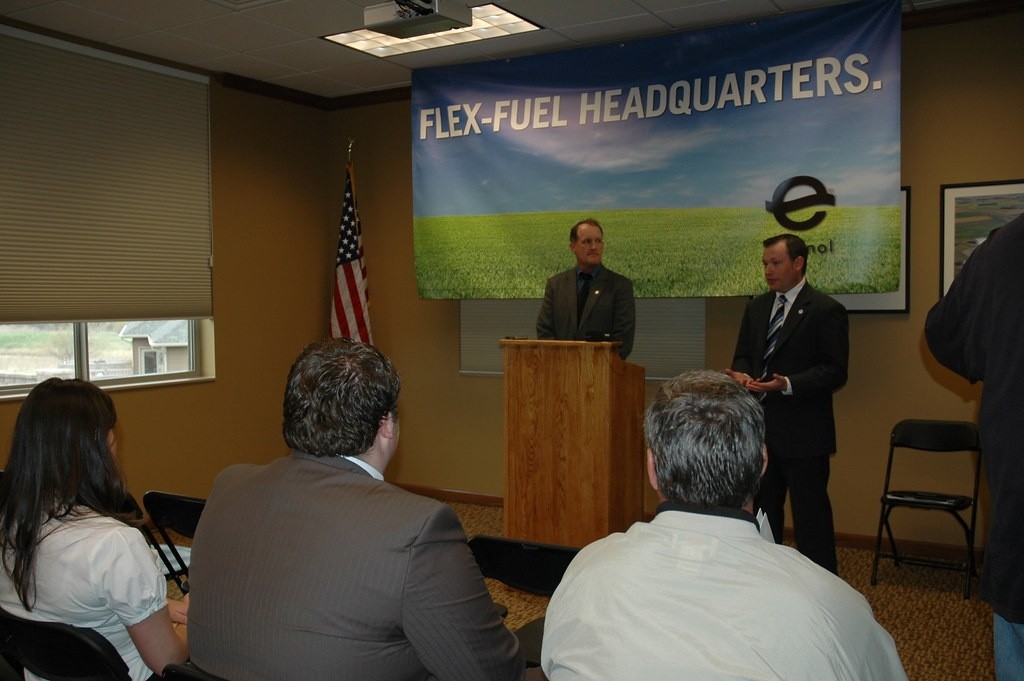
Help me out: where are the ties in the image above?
[576,272,592,332]
[758,295,789,415]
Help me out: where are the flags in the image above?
[329,159,374,348]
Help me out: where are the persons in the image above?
[534,216,636,363]
[0,377,188,681]
[725,232,851,577]
[924,214,1024,681]
[187,337,526,681]
[538,368,913,681]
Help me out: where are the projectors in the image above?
[364,0,472,39]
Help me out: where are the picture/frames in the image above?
[828,186,912,314]
[939,178,1024,300]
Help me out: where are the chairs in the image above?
[870,419,980,586]
[142,489,206,590]
[115,487,185,596]
[469,533,581,598]
[0,606,133,681]
